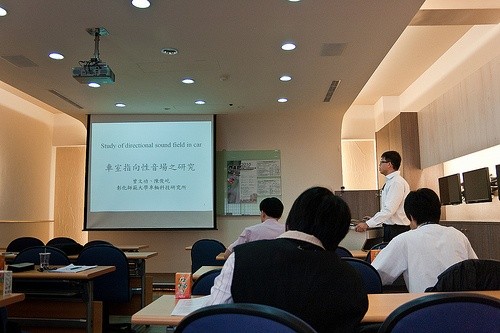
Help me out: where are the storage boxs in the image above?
[0,270,13,297]
[174,271,193,301]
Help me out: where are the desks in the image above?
[131,290,500,324]
[5,252,158,258]
[5,265,116,279]
[192,265,223,279]
[216,250,367,260]
[116,243,148,249]
[0,237,500,333]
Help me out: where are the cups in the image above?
[2,252,19,265]
[39,253,51,268]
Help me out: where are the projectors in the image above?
[72,58,116,84]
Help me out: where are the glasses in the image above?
[380,161,392,165]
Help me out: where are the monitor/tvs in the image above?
[438,173,462,205]
[463,167,492,204]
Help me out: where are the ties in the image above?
[382,183,386,190]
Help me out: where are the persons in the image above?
[371,188,479,293]
[205,187,368,333]
[223,197,285,258]
[355,151,410,242]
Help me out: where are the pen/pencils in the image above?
[71,267,82,270]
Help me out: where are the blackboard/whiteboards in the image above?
[216,149,282,216]
